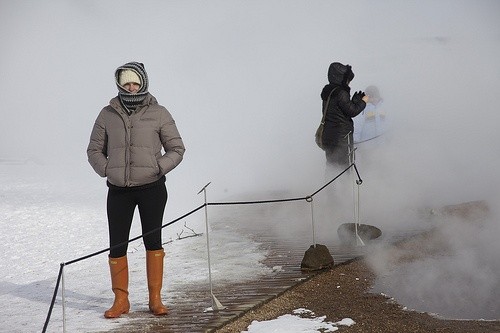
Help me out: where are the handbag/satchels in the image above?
[315,124,326,150]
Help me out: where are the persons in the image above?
[362,86,387,157]
[314,62,365,209]
[87,62,186,319]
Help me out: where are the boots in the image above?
[146,248,170,316]
[104,254,130,318]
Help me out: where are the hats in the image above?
[119,70,141,87]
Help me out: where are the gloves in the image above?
[351,91,365,104]
